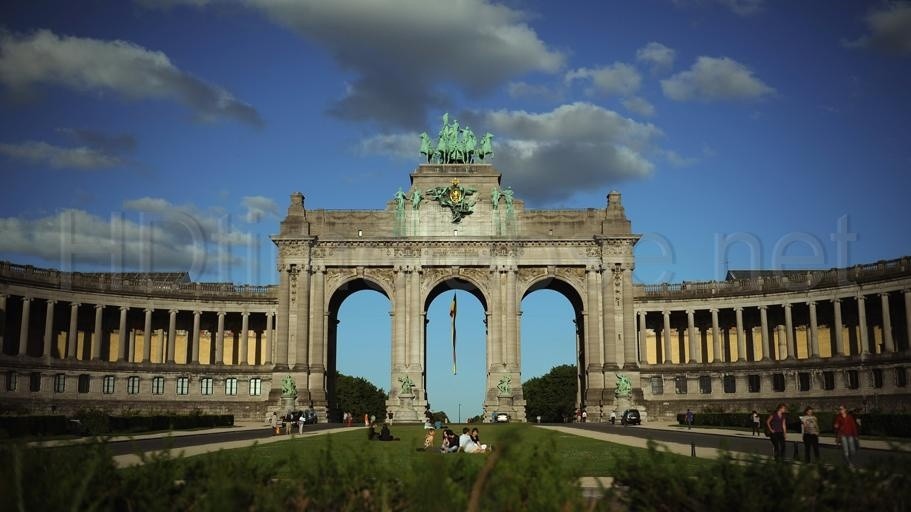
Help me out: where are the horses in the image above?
[478,132,494,164]
[437,132,453,164]
[419,132,434,164]
[455,134,475,164]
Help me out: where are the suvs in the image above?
[622,408,641,425]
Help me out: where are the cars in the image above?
[285,410,317,424]
[497,413,508,422]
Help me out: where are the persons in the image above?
[397,377,416,394]
[271,403,863,467]
[614,374,630,394]
[497,375,513,394]
[393,110,515,210]
[277,372,297,396]
